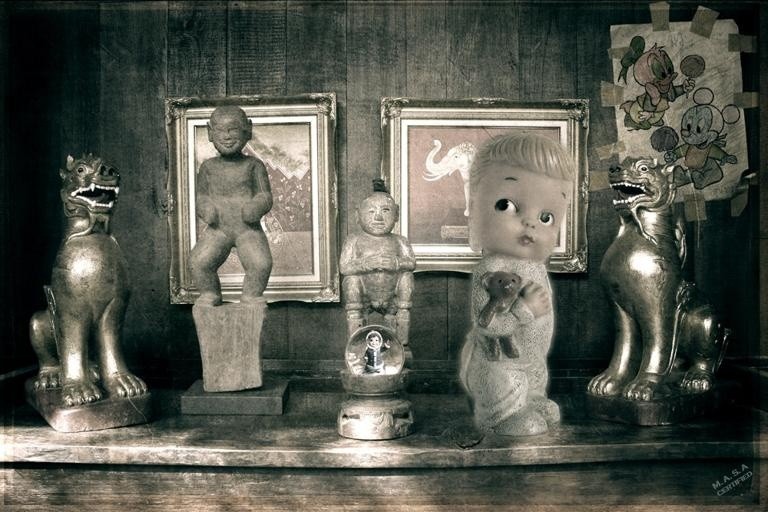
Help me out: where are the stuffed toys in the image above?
[476,270,522,361]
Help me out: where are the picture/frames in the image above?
[163,90,589,307]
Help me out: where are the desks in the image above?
[4,387,767,510]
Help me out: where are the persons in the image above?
[337,191,416,359]
[186,105,276,310]
[360,331,391,374]
[455,133,575,440]
